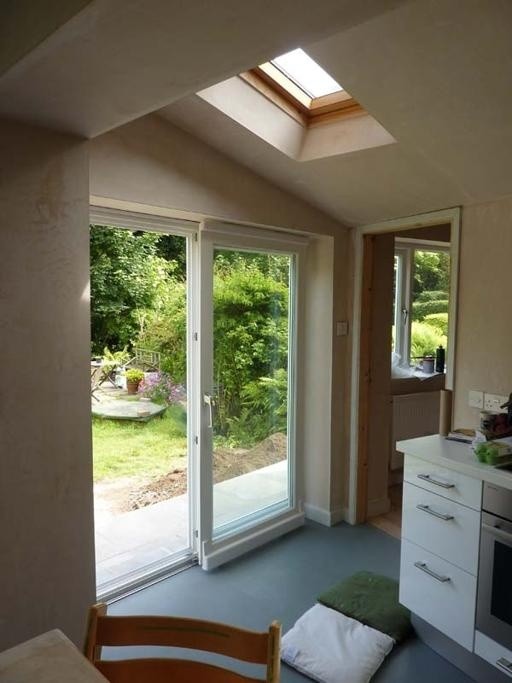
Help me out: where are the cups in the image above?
[479,410,499,433]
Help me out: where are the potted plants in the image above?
[123,369,144,393]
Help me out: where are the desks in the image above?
[0,628,111,683]
[90,359,119,402]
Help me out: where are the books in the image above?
[477,429,511,441]
[447,429,476,441]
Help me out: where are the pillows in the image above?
[318,569,410,641]
[279,602,396,683]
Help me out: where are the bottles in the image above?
[435,344,444,370]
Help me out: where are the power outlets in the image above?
[483,393,497,413]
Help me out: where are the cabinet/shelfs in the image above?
[395,431,512,683]
[390,390,441,472]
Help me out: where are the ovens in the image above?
[474,510,511,652]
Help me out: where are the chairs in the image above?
[87,603,281,683]
